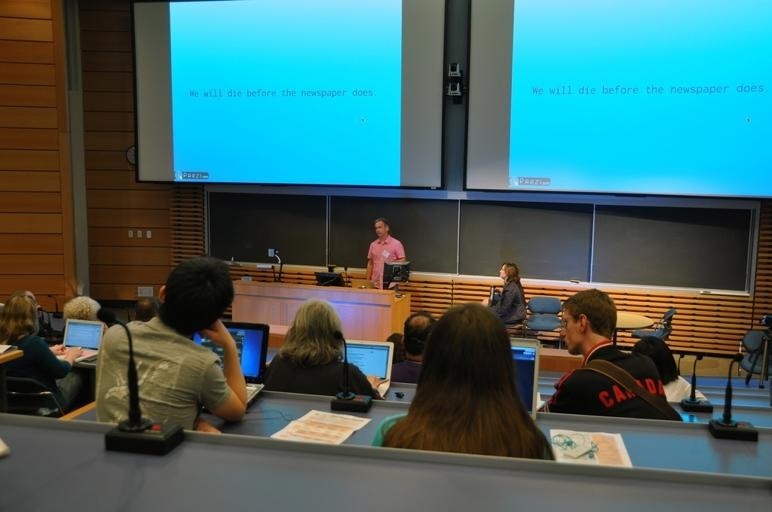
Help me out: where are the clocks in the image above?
[125,144,136,167]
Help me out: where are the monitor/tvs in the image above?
[314,271,344,286]
[384,260,411,282]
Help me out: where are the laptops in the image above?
[508,338,540,421]
[340,339,394,397]
[56,318,105,362]
[190,322,269,413]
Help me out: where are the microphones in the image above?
[37,307,56,338]
[678,352,758,441]
[95,308,183,455]
[277,262,284,283]
[331,329,373,413]
[47,295,62,319]
[345,266,347,287]
[271,265,277,282]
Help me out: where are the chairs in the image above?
[492,293,526,339]
[523,296,564,349]
[631,306,677,343]
[735,329,771,379]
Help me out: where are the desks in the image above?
[1,340,771,510]
[231,278,411,345]
[609,309,656,350]
